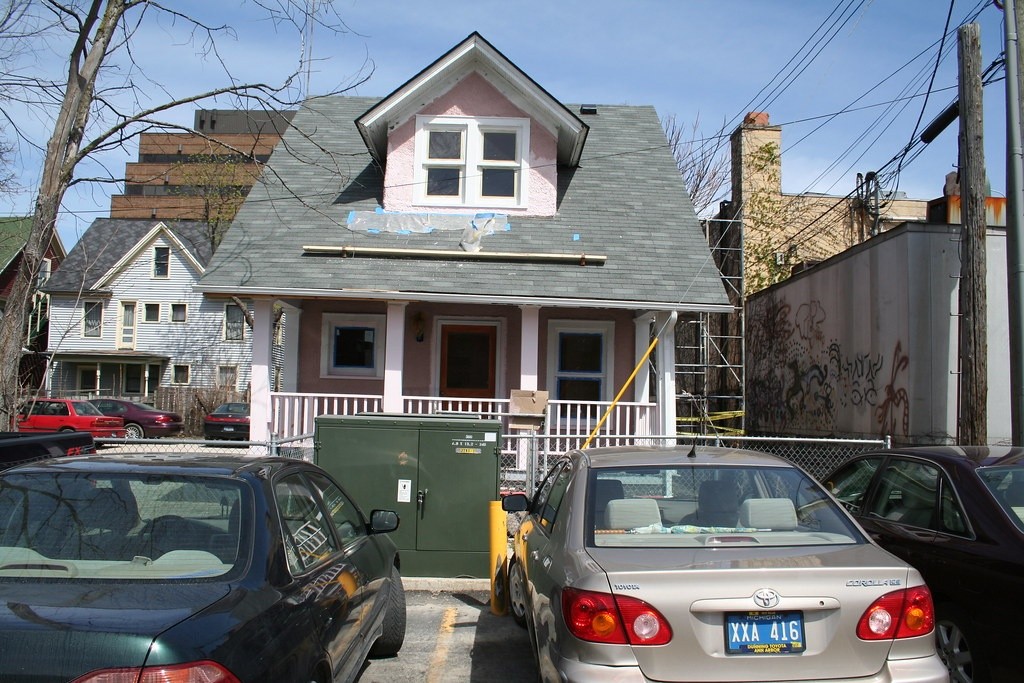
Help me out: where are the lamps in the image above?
[164,174,170,184]
[152,208,157,215]
[200,109,206,121]
[413,313,426,342]
[212,109,217,122]
[178,143,183,151]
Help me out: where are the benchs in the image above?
[0,547,228,578]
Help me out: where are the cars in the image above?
[810,443,1023,681]
[204,402,251,440]
[0,451,408,683]
[501,444,951,683]
[9,398,126,438]
[87,398,185,441]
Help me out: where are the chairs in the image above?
[594,478,624,529]
[679,480,740,529]
[885,479,938,525]
[604,497,662,531]
[1006,481,1024,507]
[742,499,801,533]
[79,485,131,533]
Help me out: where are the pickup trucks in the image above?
[0,431,99,476]
